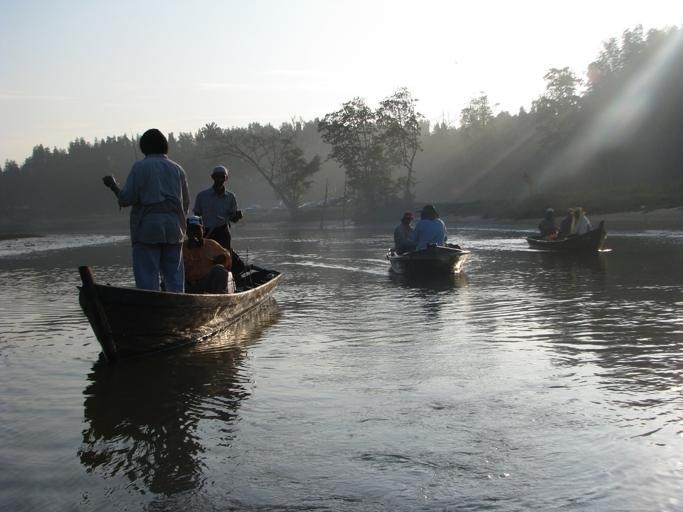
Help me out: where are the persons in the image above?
[557,207,575,237]
[424,204,447,243]
[101,127,189,294]
[181,216,232,293]
[537,207,558,240]
[191,166,243,250]
[564,206,592,241]
[393,212,415,255]
[409,207,443,252]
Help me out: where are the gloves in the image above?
[386,242,471,275]
[526,220,607,253]
[77,264,283,364]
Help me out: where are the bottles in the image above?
[184,214,205,229]
[568,207,587,217]
[209,164,229,177]
[402,211,415,221]
[414,204,438,214]
